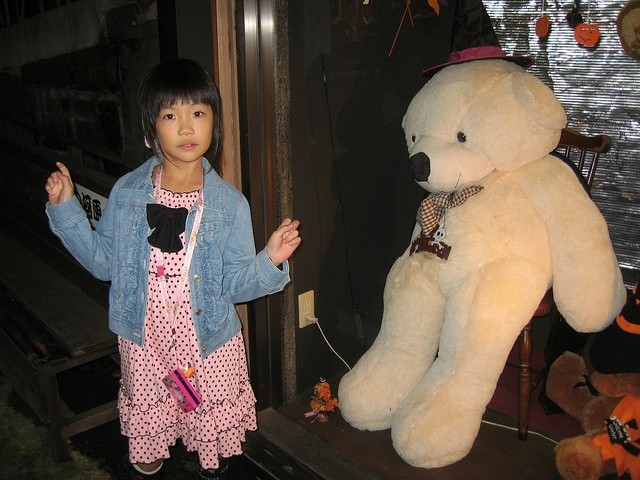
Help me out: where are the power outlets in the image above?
[298,291,315,329]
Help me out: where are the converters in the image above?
[304,314,565,446]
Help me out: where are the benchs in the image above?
[0,229,122,464]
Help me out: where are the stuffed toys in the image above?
[338,60,629,468]
[546,353,639,480]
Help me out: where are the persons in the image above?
[46,60,301,479]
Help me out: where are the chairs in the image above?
[517,131,611,441]
